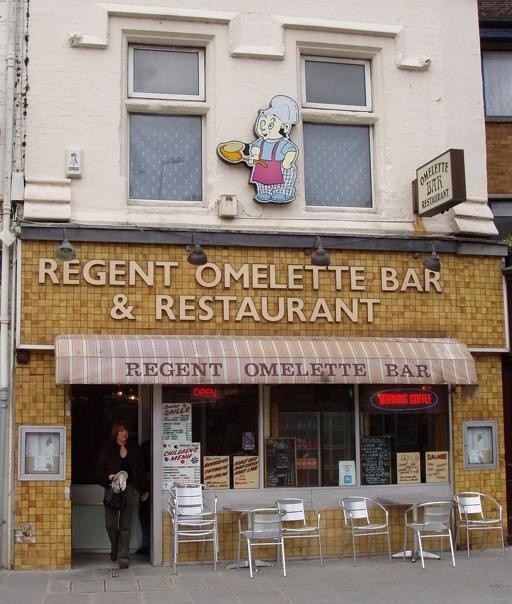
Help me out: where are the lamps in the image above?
[55,237,77,261]
[305,236,330,266]
[414,241,442,272]
[184,241,208,266]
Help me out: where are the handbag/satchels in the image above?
[103,488,126,509]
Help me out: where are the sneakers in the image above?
[255,193,296,204]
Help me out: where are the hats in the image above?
[265,95,299,126]
[112,470,128,494]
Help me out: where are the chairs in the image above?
[275,496,325,568]
[453,490,504,559]
[398,500,458,568]
[237,508,287,579]
[337,496,391,568]
[166,481,219,575]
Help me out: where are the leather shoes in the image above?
[119,558,129,568]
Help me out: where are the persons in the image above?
[137,437,152,557]
[101,422,149,567]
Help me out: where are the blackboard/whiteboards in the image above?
[361,436,392,485]
[263,437,297,487]
